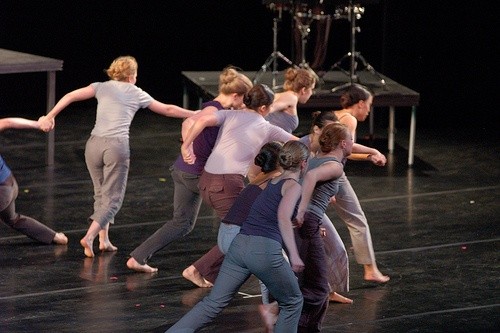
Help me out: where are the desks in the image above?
[0,48,63,166]
[182,70,420,166]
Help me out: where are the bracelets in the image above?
[367,154,373,161]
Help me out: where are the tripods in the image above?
[253,8,386,93]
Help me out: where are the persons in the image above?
[38,56,203,257]
[0,117,68,245]
[128,65,390,333]
